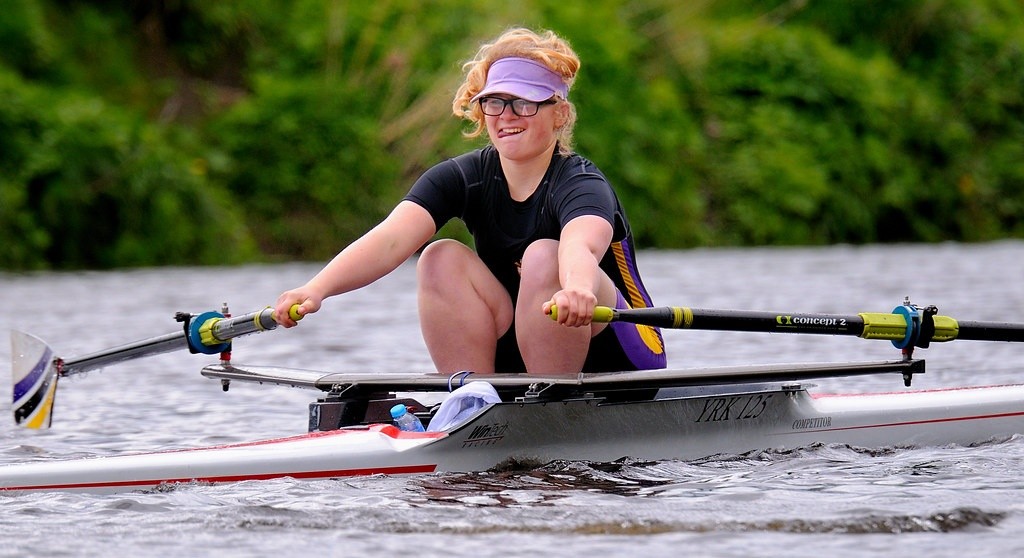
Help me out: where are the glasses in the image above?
[478,96,557,117]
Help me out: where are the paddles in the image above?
[543,298,1024,350]
[9,303,302,433]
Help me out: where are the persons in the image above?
[275,28,666,404]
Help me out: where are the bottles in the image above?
[390,404,426,432]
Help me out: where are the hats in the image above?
[469,57,570,103]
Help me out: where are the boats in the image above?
[0,374,1024,497]
